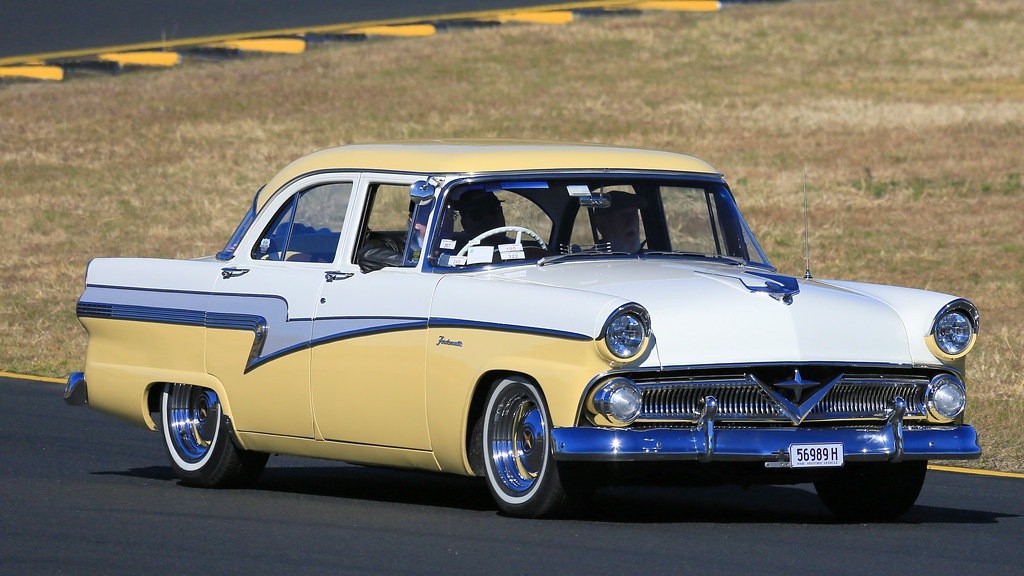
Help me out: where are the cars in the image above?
[62,137,982,517]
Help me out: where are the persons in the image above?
[590,190,658,255]
[437,190,553,267]
[357,196,458,273]
